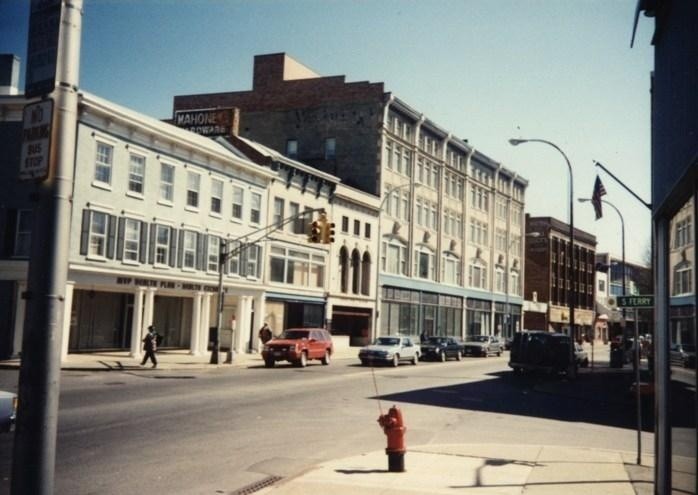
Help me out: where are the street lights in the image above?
[509,138,575,348]
[374,181,422,341]
[577,198,628,366]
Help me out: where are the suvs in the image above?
[261,327,333,368]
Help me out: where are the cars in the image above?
[420,336,466,362]
[462,335,504,358]
[358,335,422,367]
[508,330,589,381]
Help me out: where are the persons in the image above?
[259,322,272,344]
[420,330,429,343]
[141,324,157,368]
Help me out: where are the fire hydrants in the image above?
[377,405,406,472]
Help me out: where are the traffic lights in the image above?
[311,216,335,244]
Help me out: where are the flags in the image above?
[592,174,607,220]
